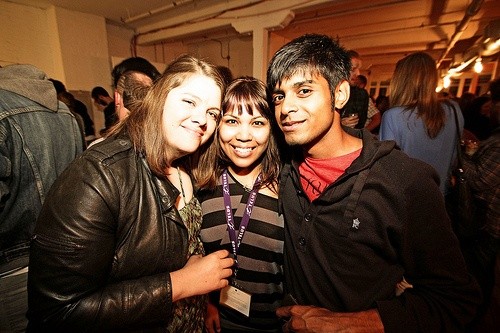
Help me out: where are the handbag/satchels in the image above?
[457,177,475,223]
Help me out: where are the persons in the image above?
[0,32,500,332]
